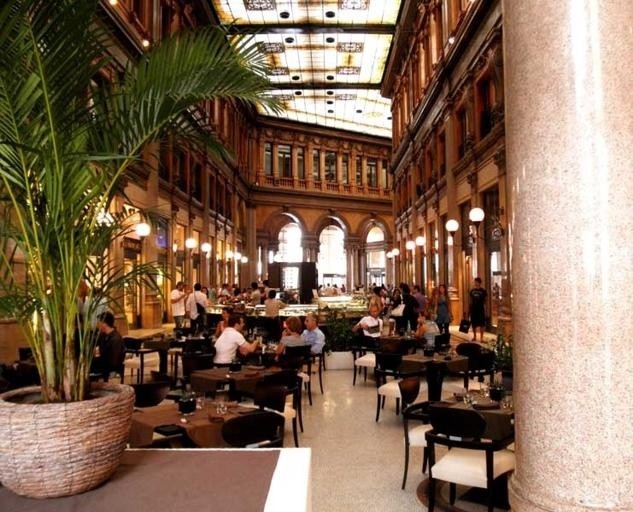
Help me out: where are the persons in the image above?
[170,276,325,373]
[92,312,125,374]
[327,276,490,350]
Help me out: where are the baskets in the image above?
[0,382,136,499]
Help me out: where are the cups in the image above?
[195,392,204,410]
[216,396,227,415]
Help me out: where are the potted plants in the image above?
[0,0,291,501]
[322,310,356,351]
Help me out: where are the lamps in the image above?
[387,235,427,265]
[224,250,233,264]
[135,220,151,245]
[201,242,212,255]
[444,217,464,247]
[185,238,197,253]
[466,206,489,242]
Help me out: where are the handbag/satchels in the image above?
[459,319,471,333]
[196,302,206,314]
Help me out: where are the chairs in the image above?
[348,322,514,512]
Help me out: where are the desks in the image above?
[0,446,312,512]
